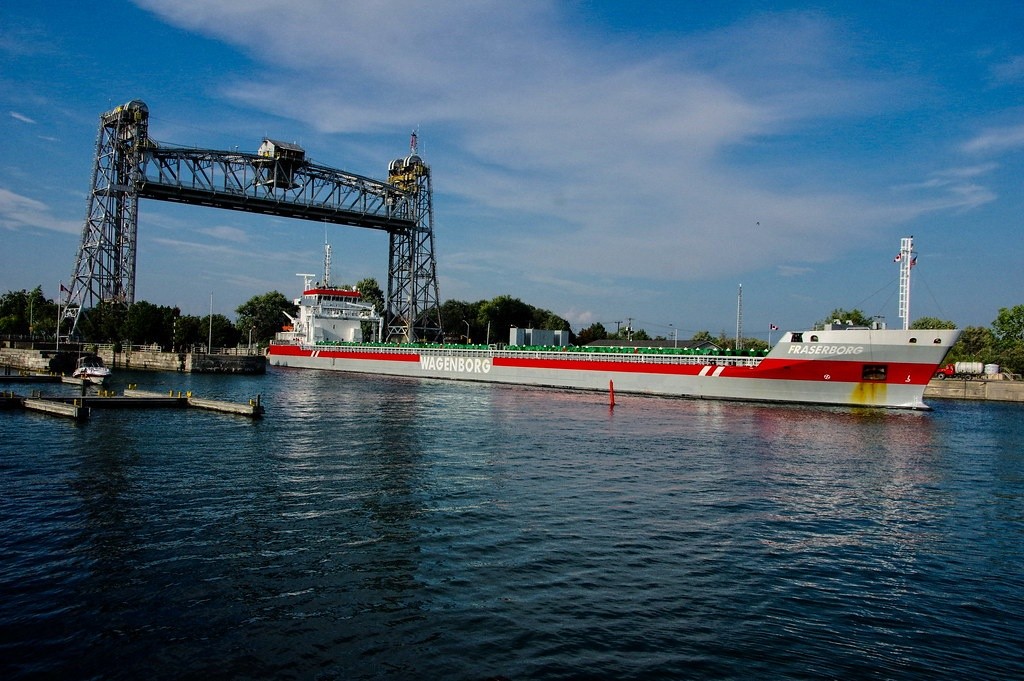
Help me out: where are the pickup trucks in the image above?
[936,360,983,380]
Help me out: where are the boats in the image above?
[73,355,113,384]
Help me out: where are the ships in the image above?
[268,236,959,411]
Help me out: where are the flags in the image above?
[893,252,901,264]
[910,255,917,270]
[61,284,72,295]
[770,323,779,331]
